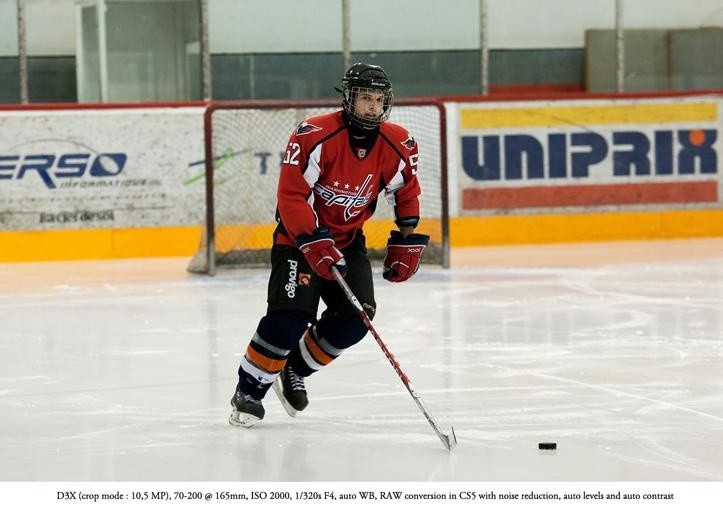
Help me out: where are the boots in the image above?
[232,383,265,420]
[280,364,309,411]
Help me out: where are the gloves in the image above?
[383,230,430,283]
[294,227,348,280]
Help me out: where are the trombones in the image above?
[331,265,457,452]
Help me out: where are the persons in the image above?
[229,62,428,420]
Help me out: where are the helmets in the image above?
[334,63,394,131]
